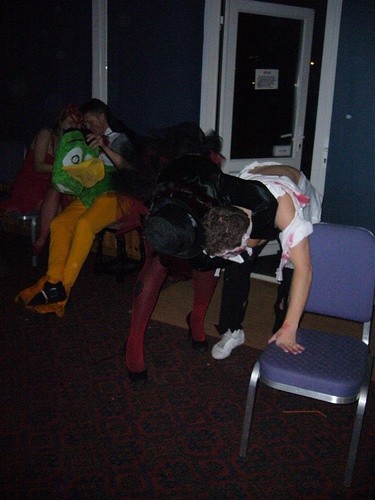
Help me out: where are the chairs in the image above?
[236,222,375,491]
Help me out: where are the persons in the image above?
[203,164,322,358]
[11,100,81,256]
[124,154,278,380]
[14,98,149,320]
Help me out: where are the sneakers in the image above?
[18,274,66,318]
[212,328,244,359]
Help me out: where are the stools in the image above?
[12,214,53,269]
[95,228,147,281]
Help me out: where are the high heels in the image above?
[30,236,47,256]
[185,311,209,352]
[122,340,149,385]
